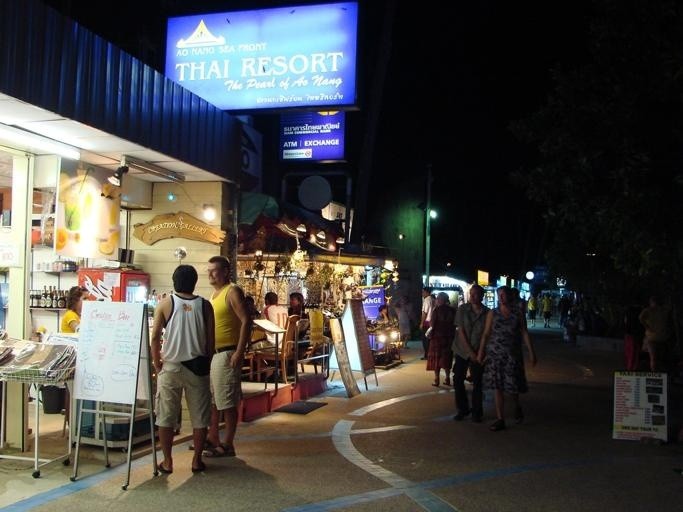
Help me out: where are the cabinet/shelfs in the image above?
[28,264,77,312]
[1,367,76,479]
[366,327,403,370]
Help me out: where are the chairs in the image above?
[238,314,330,384]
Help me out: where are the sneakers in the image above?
[514,407,523,424]
[489,420,505,431]
[454,410,470,421]
[472,415,481,423]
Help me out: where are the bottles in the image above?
[38,258,77,272]
[30,285,68,308]
[149,288,166,310]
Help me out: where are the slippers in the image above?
[192,462,205,472]
[158,461,172,474]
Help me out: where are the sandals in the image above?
[189,440,235,457]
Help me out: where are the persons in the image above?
[477,286,538,432]
[287,293,310,338]
[189,256,251,459]
[375,305,400,361]
[452,284,495,422]
[149,264,215,474]
[617,296,646,372]
[426,292,456,387]
[245,296,260,341]
[419,287,435,360]
[638,293,673,372]
[524,292,582,330]
[61,285,90,332]
[251,292,289,351]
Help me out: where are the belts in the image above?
[214,345,236,354]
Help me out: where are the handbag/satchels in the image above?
[425,327,433,339]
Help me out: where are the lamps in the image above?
[107,162,129,187]
[296,219,346,244]
